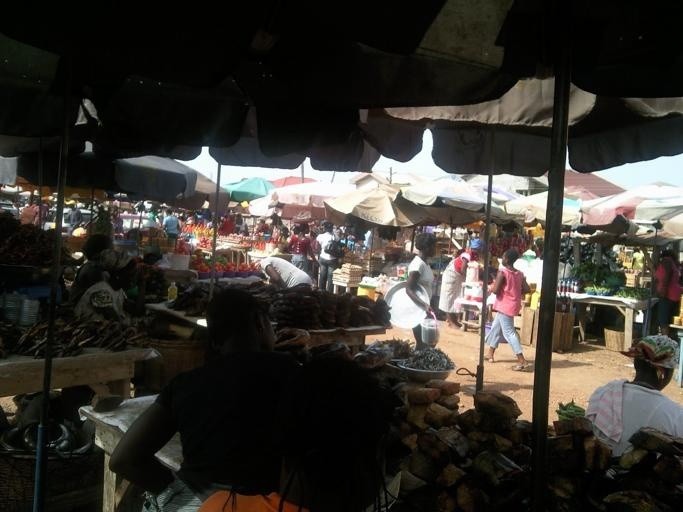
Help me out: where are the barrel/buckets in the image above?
[420,312,440,345]
[2,297,39,326]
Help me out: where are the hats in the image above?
[619,335,680,369]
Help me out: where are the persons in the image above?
[21,190,683,374]
[105,287,333,510]
[581,333,682,464]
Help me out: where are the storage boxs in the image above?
[521,305,575,355]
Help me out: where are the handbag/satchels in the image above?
[326,241,343,257]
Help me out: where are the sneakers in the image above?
[484,356,529,371]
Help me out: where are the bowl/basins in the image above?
[396,358,457,383]
[384,281,430,330]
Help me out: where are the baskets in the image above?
[604,327,637,352]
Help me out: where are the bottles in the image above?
[168,282,177,303]
[558,279,580,293]
